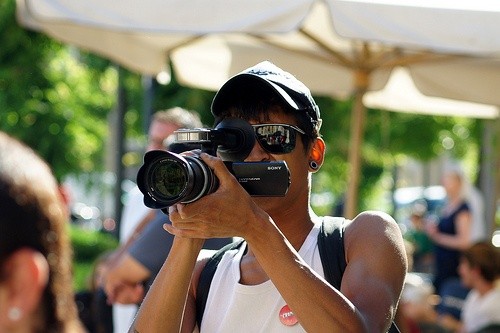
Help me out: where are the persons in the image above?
[399,160,500,333]
[94,61,408,333]
[0,132,83,333]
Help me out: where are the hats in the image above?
[211,61,320,128]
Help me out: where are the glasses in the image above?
[251,123,310,155]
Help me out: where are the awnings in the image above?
[16,1,500,118]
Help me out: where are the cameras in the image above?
[137,116,291,216]
